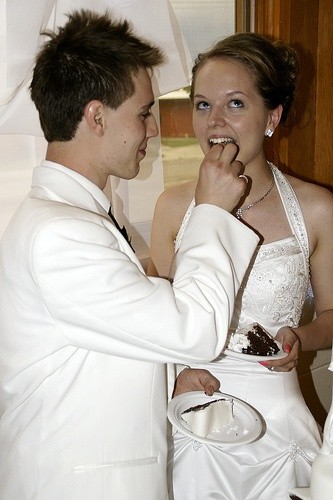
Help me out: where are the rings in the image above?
[270,366,275,371]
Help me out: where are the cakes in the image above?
[225,322,281,357]
[181,396,235,437]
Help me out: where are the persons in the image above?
[0,8,263,500]
[145,34,332,500]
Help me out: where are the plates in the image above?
[166,390,263,447]
[221,338,288,362]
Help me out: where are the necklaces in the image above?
[233,176,276,220]
